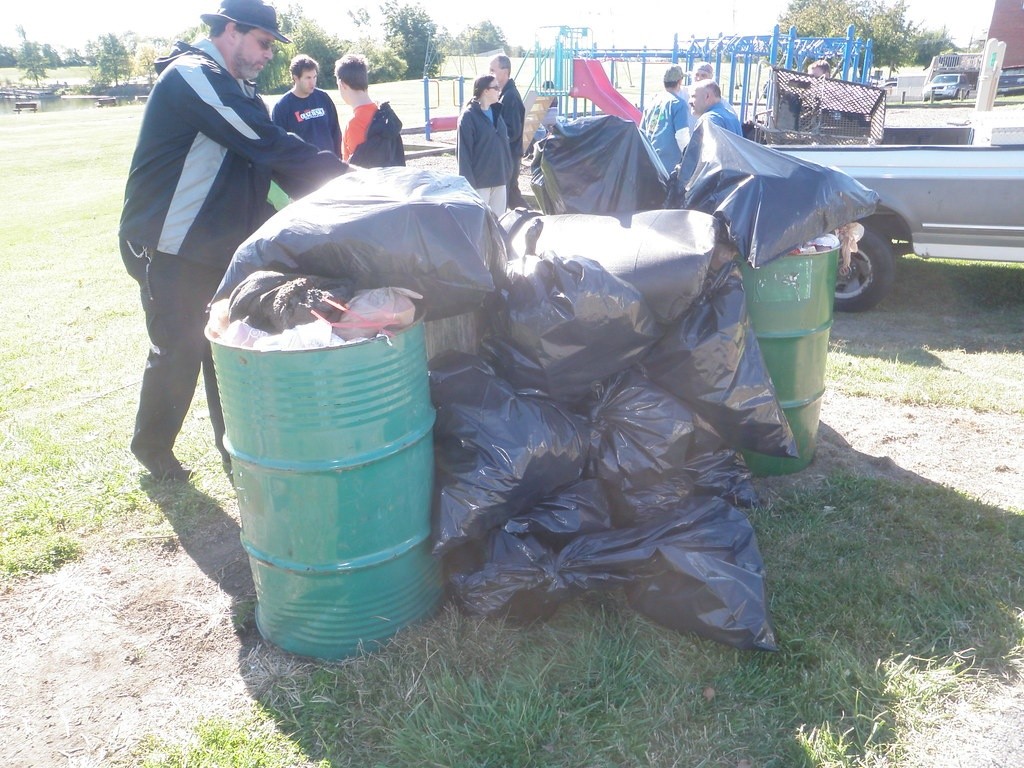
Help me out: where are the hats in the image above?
[200,0,292,44]
[661,64,689,82]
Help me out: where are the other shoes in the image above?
[130,444,192,480]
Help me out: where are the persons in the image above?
[639,66,692,175]
[334,54,406,168]
[271,53,342,159]
[118,0,368,480]
[455,76,514,219]
[687,79,743,137]
[807,59,831,79]
[488,55,533,211]
[523,81,558,159]
[674,61,737,137]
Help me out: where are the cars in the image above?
[921,73,971,101]
[997,63,1024,97]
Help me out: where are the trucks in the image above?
[759,127,1024,309]
[876,74,926,100]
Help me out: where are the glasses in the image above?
[486,86,499,91]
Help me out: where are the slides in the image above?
[569,59,643,127]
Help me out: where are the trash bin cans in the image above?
[203,194,844,667]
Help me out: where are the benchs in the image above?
[97,98,116,108]
[13,103,37,114]
